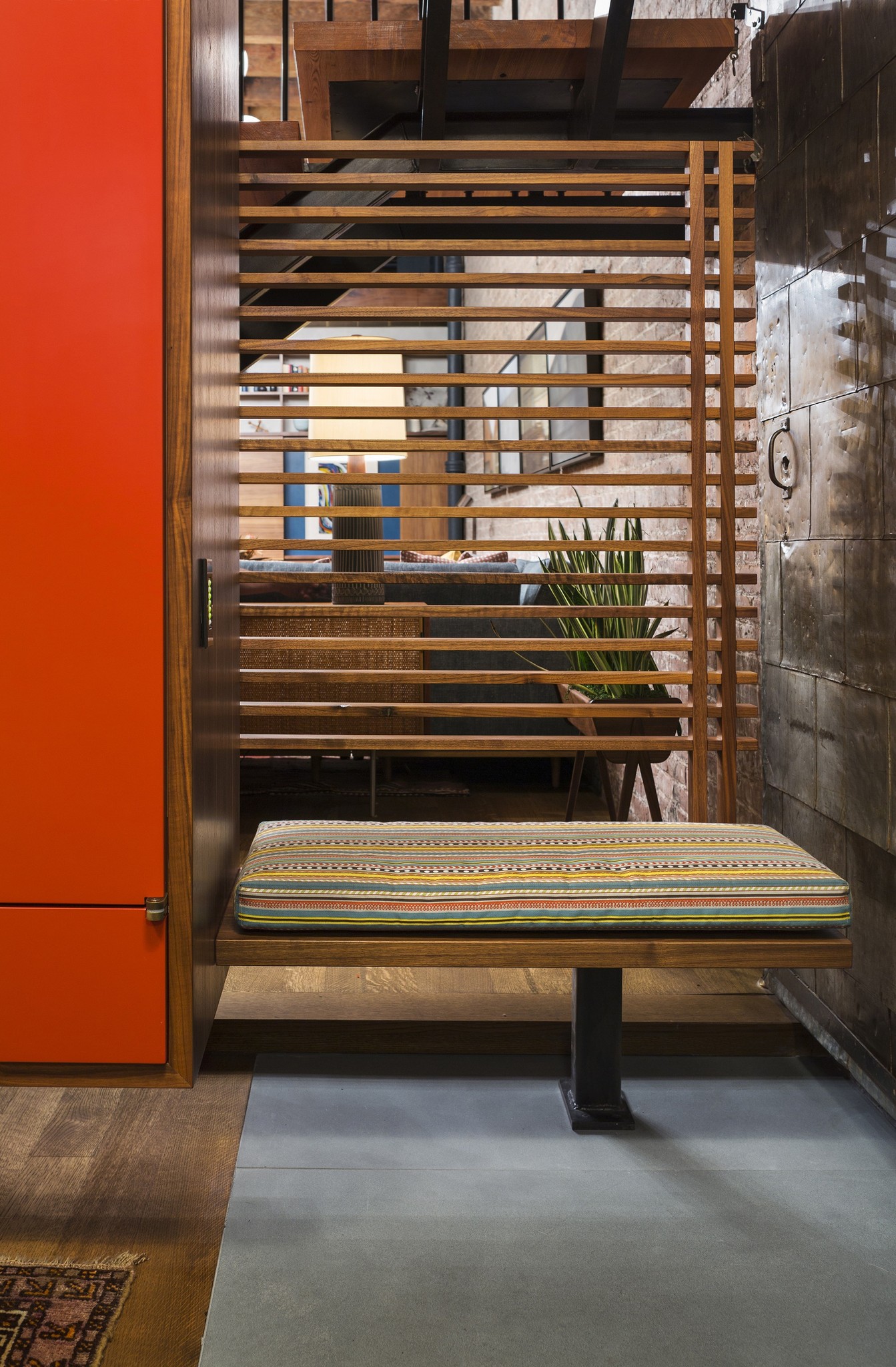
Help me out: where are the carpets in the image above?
[0,1250,149,1367]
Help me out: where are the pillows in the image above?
[400,550,516,575]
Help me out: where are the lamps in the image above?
[309,337,408,603]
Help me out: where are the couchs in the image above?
[239,559,578,789]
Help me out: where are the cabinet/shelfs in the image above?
[240,353,308,437]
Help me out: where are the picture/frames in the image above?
[480,269,604,493]
[304,451,379,539]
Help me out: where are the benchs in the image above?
[212,819,855,1135]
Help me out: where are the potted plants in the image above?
[490,482,685,822]
